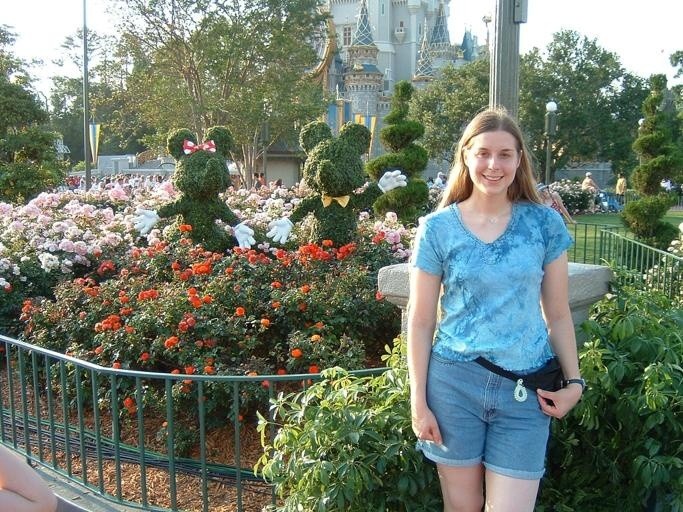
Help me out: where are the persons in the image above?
[580,171,600,193]
[438,174,446,185]
[253,172,261,189]
[406,109,587,512]
[259,172,265,186]
[615,172,627,207]
[0,448,59,512]
[65,172,165,194]
[535,182,576,225]
[426,176,434,183]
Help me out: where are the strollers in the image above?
[597,187,621,216]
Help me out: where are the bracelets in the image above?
[562,377,586,390]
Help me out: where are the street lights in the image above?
[544,102,558,185]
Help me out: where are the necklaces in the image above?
[465,198,512,224]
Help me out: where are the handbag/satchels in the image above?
[473,355,566,416]
